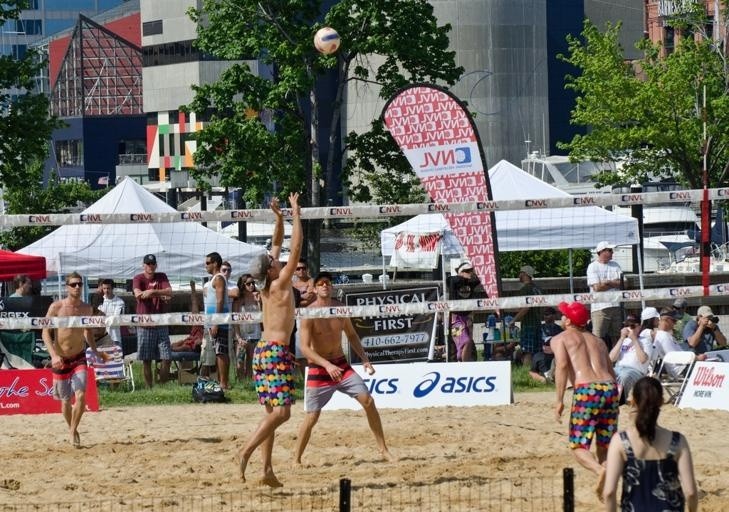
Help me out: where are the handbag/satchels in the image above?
[193,379,224,402]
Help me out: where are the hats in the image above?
[144,254,156,264]
[697,305,713,318]
[558,302,590,327]
[518,266,534,277]
[673,298,687,308]
[248,254,267,290]
[459,262,474,274]
[313,271,332,282]
[623,307,683,325]
[597,241,616,253]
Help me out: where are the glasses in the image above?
[222,268,231,273]
[296,266,306,271]
[317,280,331,286]
[67,282,83,288]
[206,261,211,265]
[268,255,274,267]
[244,281,255,286]
[624,323,636,329]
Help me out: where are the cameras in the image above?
[627,324,636,329]
[707,315,719,324]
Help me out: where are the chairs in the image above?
[86,344,135,394]
[655,350,696,406]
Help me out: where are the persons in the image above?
[585,240,629,348]
[600,375,700,512]
[238,192,304,486]
[231,272,263,382]
[292,255,317,306]
[203,251,230,390]
[89,276,103,315]
[527,355,556,386]
[8,272,33,298]
[198,260,241,378]
[531,306,557,369]
[169,279,205,352]
[41,270,106,447]
[491,315,524,363]
[653,304,707,380]
[607,313,652,400]
[678,305,727,353]
[438,276,450,349]
[548,302,622,502]
[97,279,126,358]
[637,305,661,342]
[132,254,173,386]
[507,263,543,370]
[292,272,395,466]
[447,261,480,360]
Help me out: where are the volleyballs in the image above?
[314,27,340,55]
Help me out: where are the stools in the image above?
[156,351,202,375]
